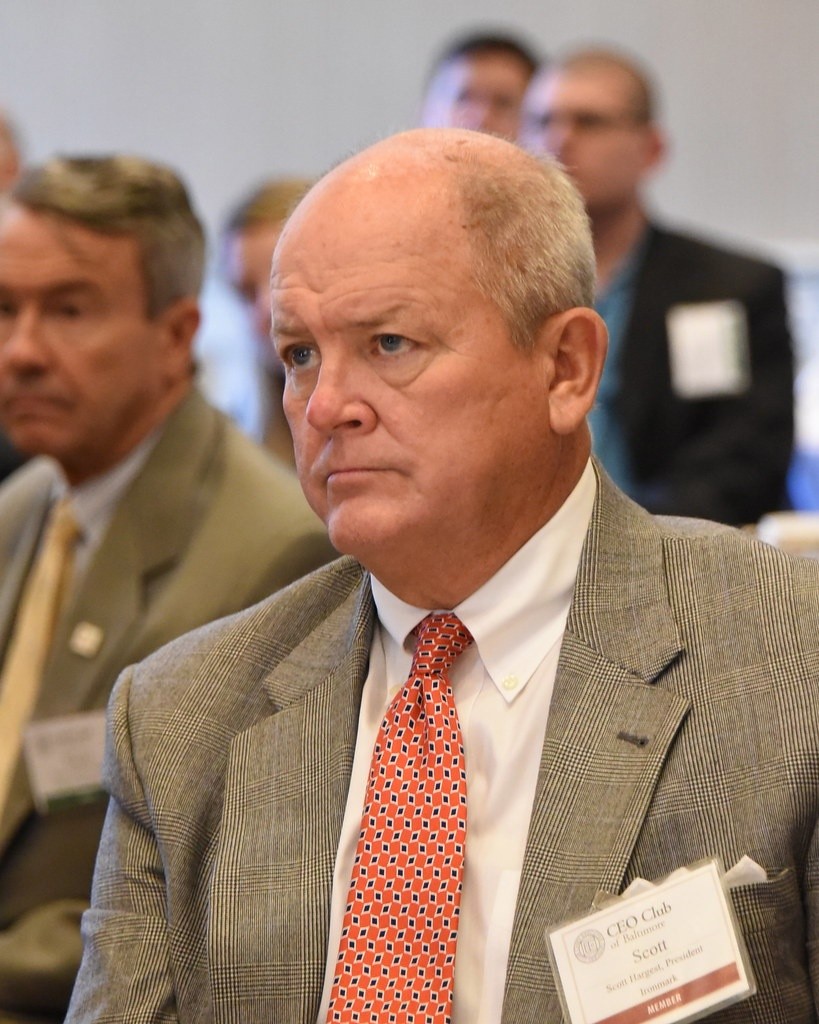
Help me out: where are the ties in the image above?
[0,502,81,855]
[325,612,481,1024]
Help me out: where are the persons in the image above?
[0,156,340,1024]
[510,49,794,523]
[217,174,337,471]
[60,128,819,1024]
[420,35,536,143]
